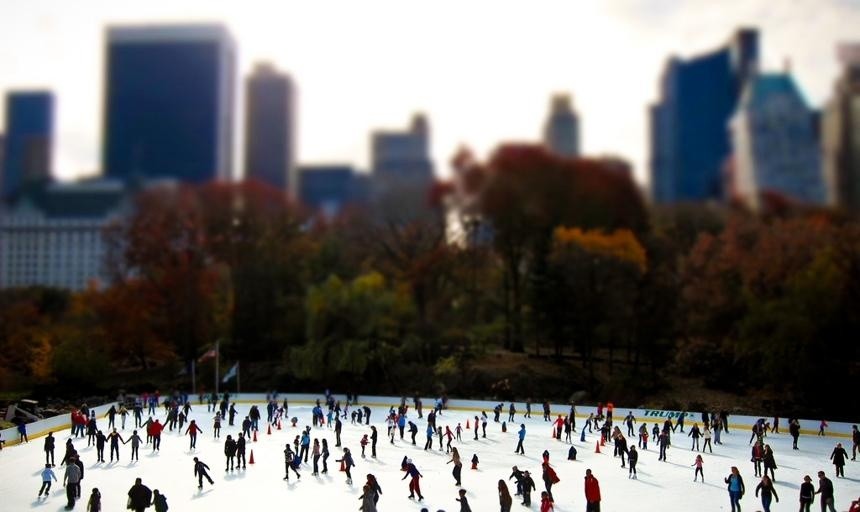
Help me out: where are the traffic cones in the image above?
[293,419,296,426]
[267,424,272,434]
[552,426,557,438]
[322,415,325,424]
[595,432,607,453]
[253,429,258,441]
[471,459,478,469]
[466,419,470,429]
[248,449,255,464]
[277,419,281,430]
[339,455,346,471]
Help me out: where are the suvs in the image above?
[18,398,40,416]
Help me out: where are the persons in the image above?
[2,380,860,512]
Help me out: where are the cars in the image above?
[40,408,62,418]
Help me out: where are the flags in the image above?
[198,343,218,362]
[177,361,201,376]
[218,365,237,383]
